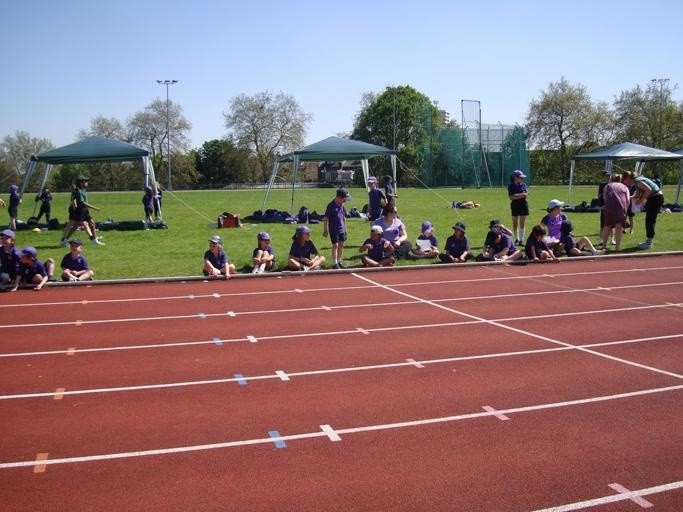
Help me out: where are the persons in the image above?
[598,171,666,250]
[452,200,480,209]
[412,221,439,259]
[508,170,530,246]
[298,207,308,221]
[251,232,275,274]
[439,221,473,263]
[525,198,606,264]
[287,225,326,273]
[323,191,353,270]
[0,174,106,293]
[142,185,155,223]
[202,235,238,280]
[359,174,411,267]
[476,219,522,262]
[151,181,162,220]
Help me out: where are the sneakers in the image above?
[599,240,603,245]
[593,250,608,255]
[610,240,617,245]
[60,239,70,248]
[581,251,593,256]
[90,235,105,246]
[333,262,347,269]
[639,243,651,249]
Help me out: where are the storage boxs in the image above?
[218,214,240,228]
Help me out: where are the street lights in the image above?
[649,77,669,177]
[384,85,403,150]
[155,79,179,191]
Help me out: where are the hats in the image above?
[75,176,90,185]
[208,236,223,245]
[336,188,353,201]
[296,227,312,233]
[1,230,15,238]
[10,185,16,191]
[15,247,37,256]
[548,199,564,209]
[422,221,432,234]
[561,220,573,232]
[371,225,384,234]
[368,177,377,184]
[452,222,465,232]
[489,220,501,228]
[257,232,271,239]
[513,170,527,178]
[68,239,82,245]
